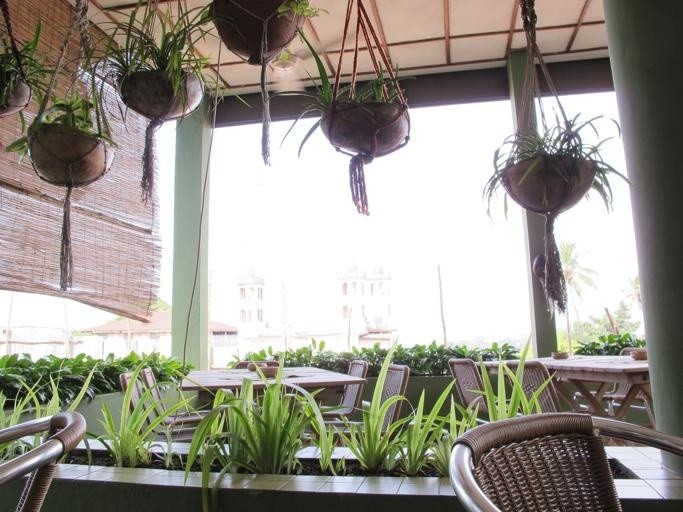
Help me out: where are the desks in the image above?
[545,358,649,420]
[471,357,595,410]
[173,364,368,400]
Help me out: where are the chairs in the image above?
[119,371,197,443]
[504,360,564,416]
[139,366,213,426]
[321,362,413,439]
[449,358,518,415]
[573,345,655,431]
[446,411,683,511]
[317,358,369,418]
[1,408,89,512]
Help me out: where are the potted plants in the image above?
[4,84,122,191]
[265,23,419,163]
[61,1,256,131]
[480,107,636,217]
[0,16,59,125]
[196,0,330,64]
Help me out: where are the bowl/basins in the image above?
[630,350,647,360]
[551,351,569,360]
[256,367,279,378]
[247,363,267,371]
